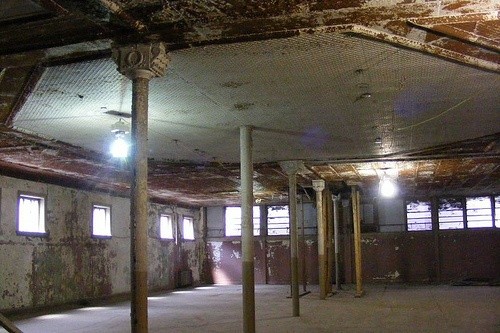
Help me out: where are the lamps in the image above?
[109,121,132,159]
